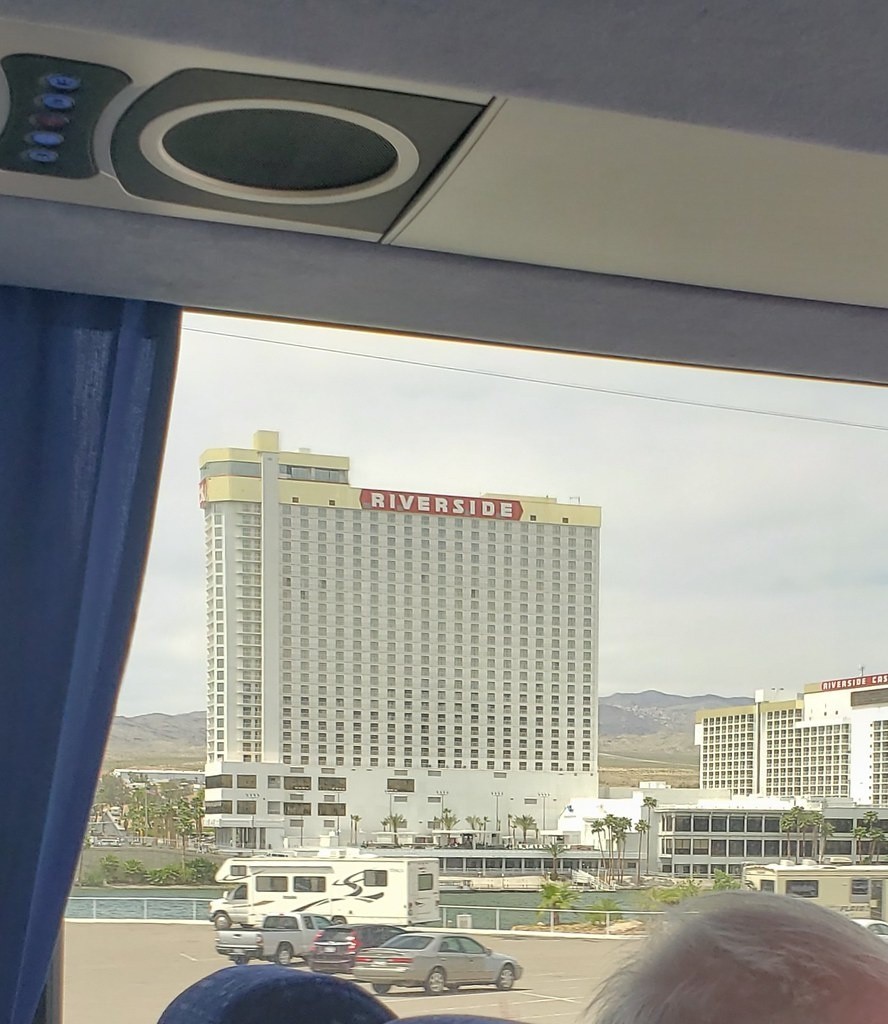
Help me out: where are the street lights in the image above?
[538,792,550,844]
[384,788,399,832]
[245,793,261,847]
[437,790,448,829]
[332,786,345,836]
[491,791,503,831]
[294,786,310,845]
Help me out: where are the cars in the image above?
[353,932,523,995]
[850,918,888,950]
[308,924,410,975]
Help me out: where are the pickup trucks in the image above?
[213,912,336,967]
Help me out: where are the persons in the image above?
[575,890,888,1024]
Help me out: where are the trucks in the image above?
[207,848,441,929]
[740,857,888,922]
